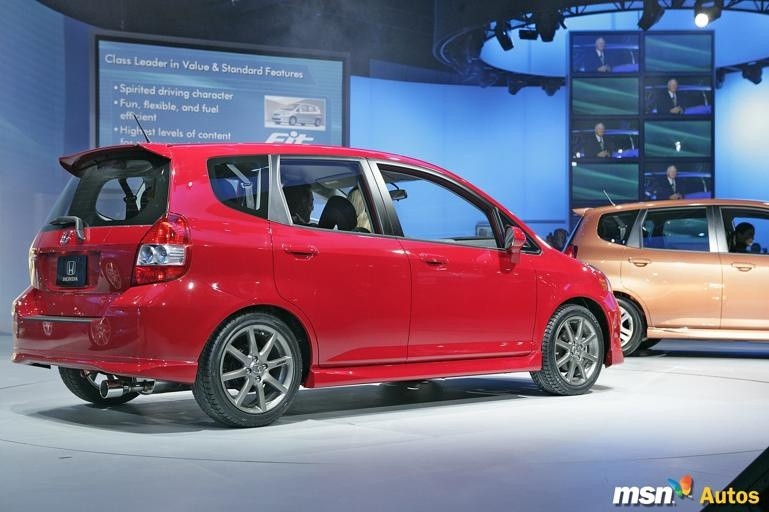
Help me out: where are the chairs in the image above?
[316,195,369,232]
[217,177,237,205]
[138,186,150,211]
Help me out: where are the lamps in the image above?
[450,0,769,97]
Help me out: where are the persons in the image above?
[282,181,317,227]
[582,122,610,158]
[731,221,754,252]
[550,229,567,251]
[751,243,760,253]
[655,165,684,200]
[655,77,685,115]
[583,36,611,73]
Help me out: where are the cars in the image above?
[645,85,711,115]
[572,44,639,72]
[11,142,769,428]
[272,103,321,126]
